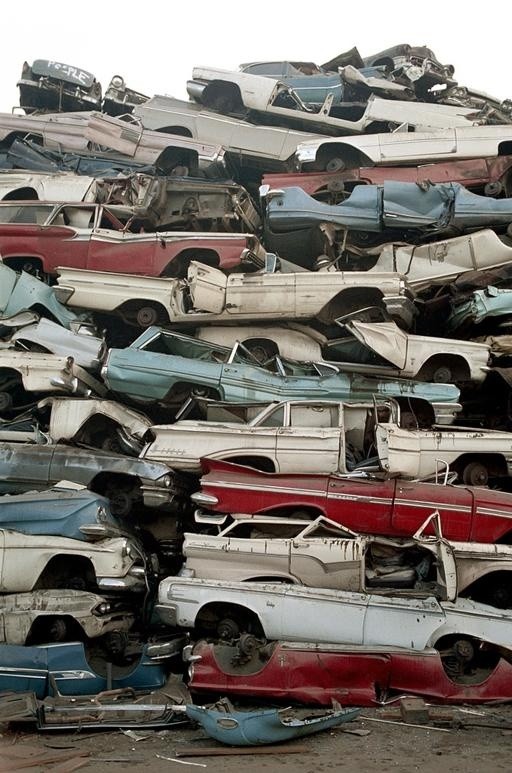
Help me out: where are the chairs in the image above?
[365,563,415,587]
[345,439,379,472]
